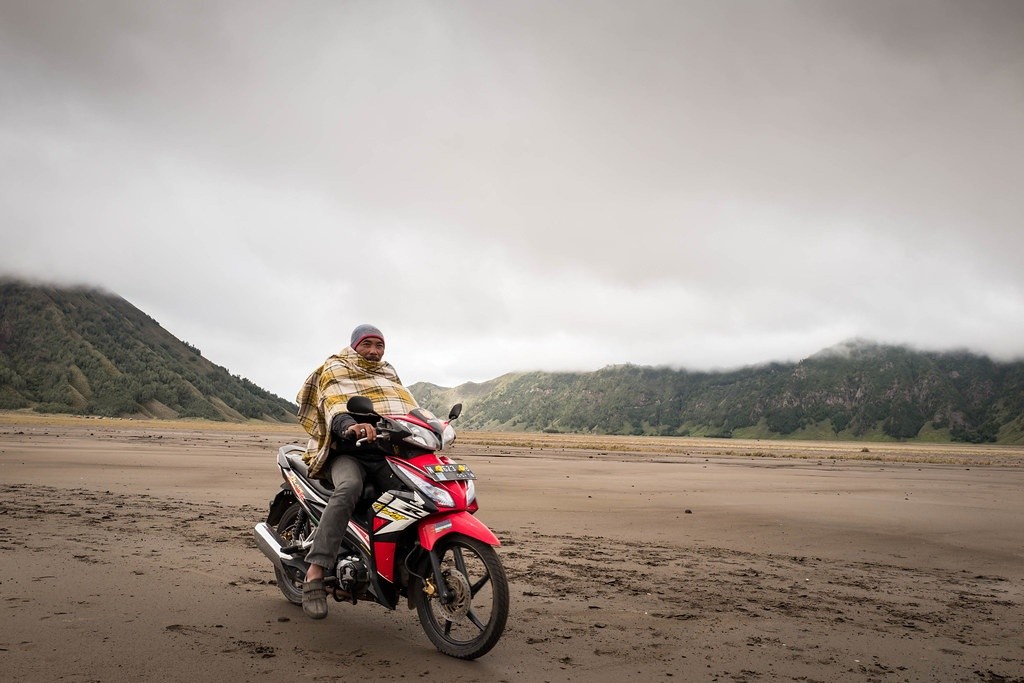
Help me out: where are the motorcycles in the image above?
[254,396,511,661]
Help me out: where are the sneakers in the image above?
[301,575,328,618]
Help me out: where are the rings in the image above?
[360,429,365,432]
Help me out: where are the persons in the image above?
[296,324,457,618]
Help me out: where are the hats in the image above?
[350,324,385,350]
